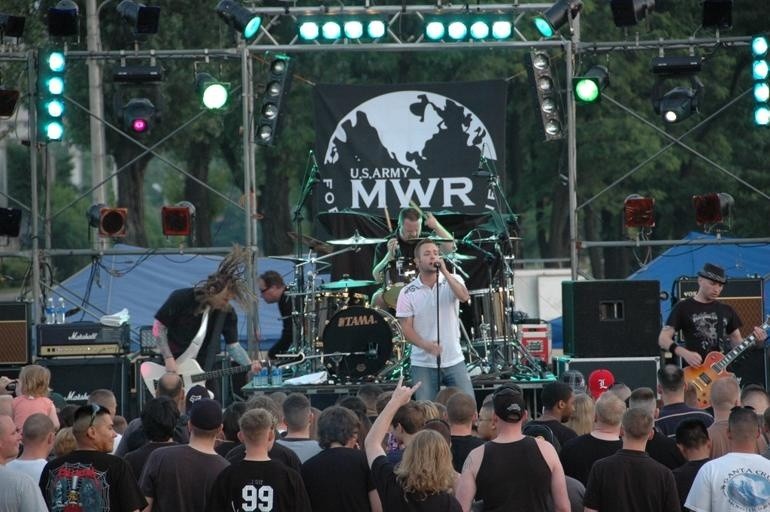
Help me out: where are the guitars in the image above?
[682,315,770,410]
[140,352,305,401]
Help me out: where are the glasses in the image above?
[85,401,102,427]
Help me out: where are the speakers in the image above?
[135,354,232,418]
[0,302,32,367]
[34,357,132,423]
[562,278,662,358]
[676,279,768,396]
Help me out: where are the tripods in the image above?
[464,243,546,382]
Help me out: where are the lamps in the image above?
[0,0,770,254]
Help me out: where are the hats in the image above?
[699,264,729,284]
[190,399,222,430]
[491,385,524,421]
[589,368,615,398]
[184,385,213,408]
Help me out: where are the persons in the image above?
[393,237,477,413]
[1,344,770,512]
[369,210,454,312]
[154,244,262,405]
[658,262,768,385]
[257,271,307,362]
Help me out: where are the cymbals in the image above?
[447,252,476,260]
[287,231,333,253]
[269,255,329,265]
[327,236,388,245]
[404,237,453,242]
[470,236,522,241]
[322,280,381,288]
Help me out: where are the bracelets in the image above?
[670,341,676,353]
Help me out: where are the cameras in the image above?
[5,381,17,391]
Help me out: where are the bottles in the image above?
[55,297,65,323]
[253,366,283,386]
[46,298,55,325]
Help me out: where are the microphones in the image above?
[479,143,485,171]
[310,150,321,180]
[434,261,442,268]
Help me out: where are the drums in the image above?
[459,287,513,348]
[322,305,406,381]
[329,293,369,315]
[382,257,418,311]
